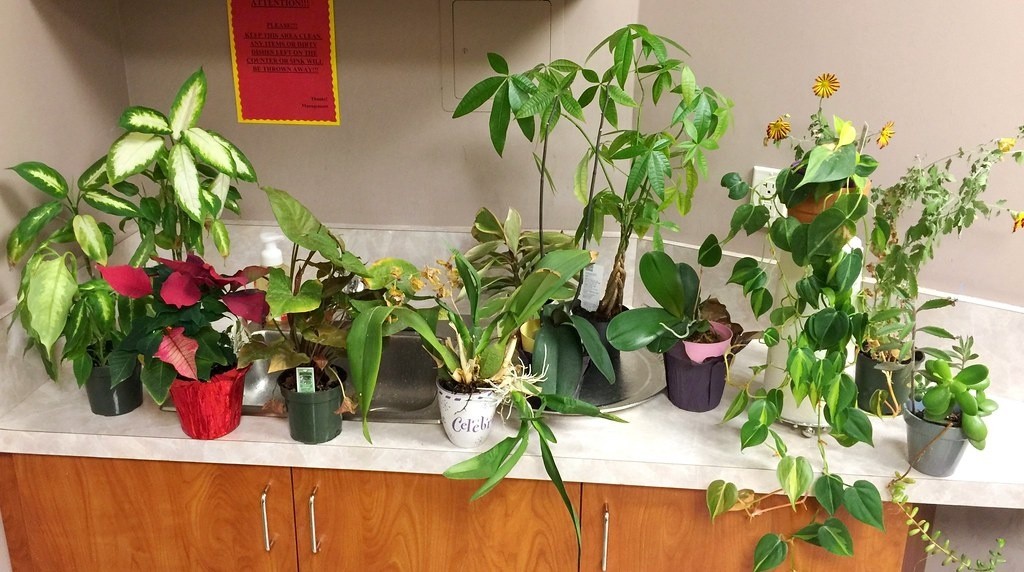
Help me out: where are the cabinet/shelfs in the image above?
[0,453,583,570]
[579,482,913,571]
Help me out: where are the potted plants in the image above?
[2,25,1024,570]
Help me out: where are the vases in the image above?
[857,338,926,416]
[787,180,870,229]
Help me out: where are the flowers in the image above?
[863,126,1024,366]
[697,70,898,572]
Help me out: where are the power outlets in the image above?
[750,166,787,229]
